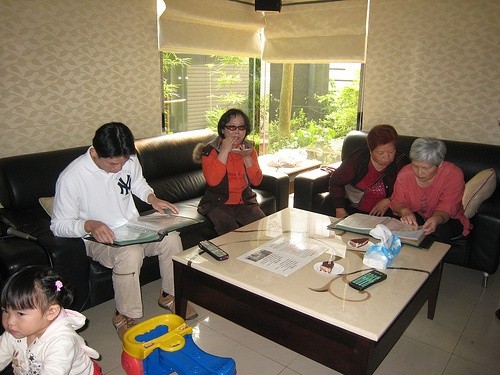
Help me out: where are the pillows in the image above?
[39,197,54,217]
[462,168,496,218]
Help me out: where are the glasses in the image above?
[224,125,248,131]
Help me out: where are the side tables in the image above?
[257,153,323,194]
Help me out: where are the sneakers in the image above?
[112,307,137,342]
[157,288,198,320]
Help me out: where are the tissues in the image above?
[362,223,401,272]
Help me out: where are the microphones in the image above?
[0,221,39,241]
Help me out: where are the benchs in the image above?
[293,130,500,288]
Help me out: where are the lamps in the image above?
[255,0,282,13]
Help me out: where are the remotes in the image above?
[349,269,387,291]
[199,239,229,261]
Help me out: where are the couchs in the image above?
[0,128,289,313]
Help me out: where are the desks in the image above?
[172,207,451,375]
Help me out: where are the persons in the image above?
[193,108,266,237]
[388,136,473,249]
[0,265,102,375]
[322,124,406,218]
[50,122,199,342]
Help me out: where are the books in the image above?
[84,212,205,248]
[326,212,437,251]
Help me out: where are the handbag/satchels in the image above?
[345,152,403,208]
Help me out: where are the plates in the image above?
[313,262,345,275]
[231,148,250,151]
